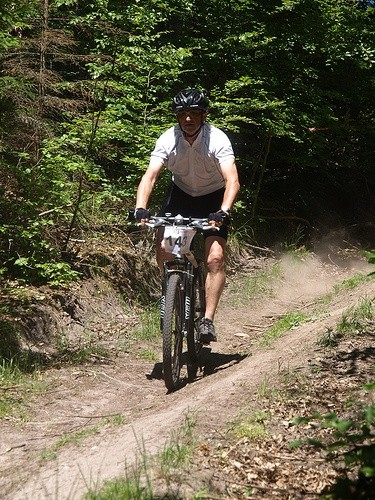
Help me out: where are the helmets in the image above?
[172,88,210,113]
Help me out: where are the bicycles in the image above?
[125,206,231,391]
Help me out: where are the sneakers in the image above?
[196,318,218,342]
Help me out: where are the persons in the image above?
[134,89,240,343]
